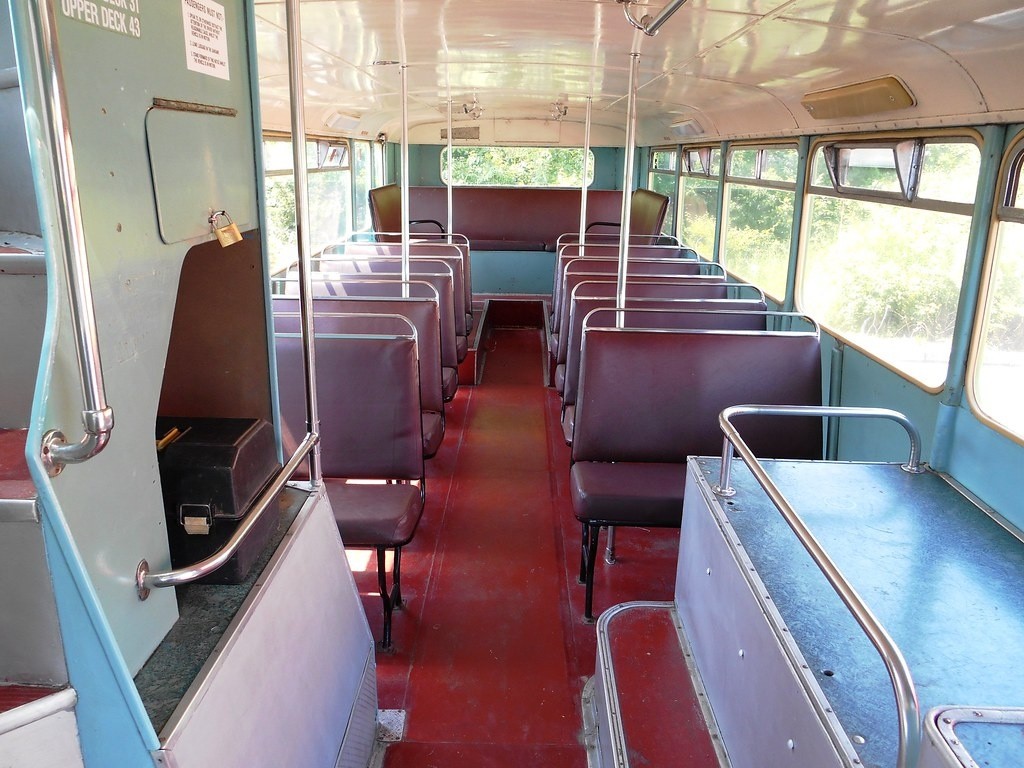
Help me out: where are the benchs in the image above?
[268,184,823,650]
